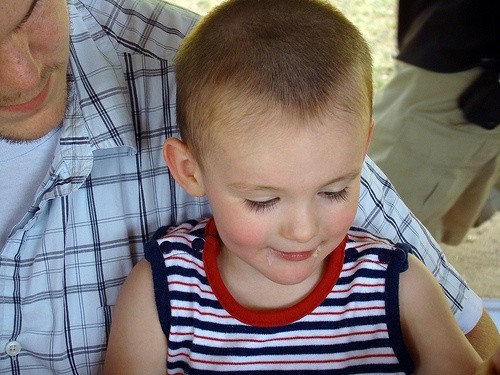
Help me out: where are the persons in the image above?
[103,0,500,375]
[366,0,500,258]
[0,0,500,375]
